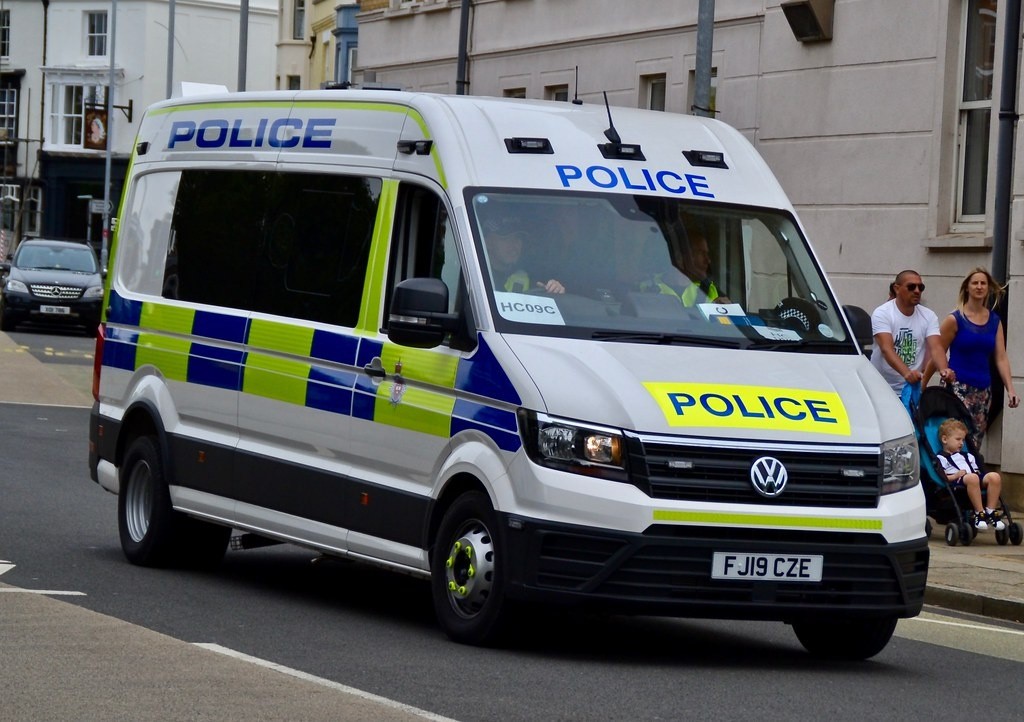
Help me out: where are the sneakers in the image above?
[985,510,1005,530]
[974,511,988,529]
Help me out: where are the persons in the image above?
[936,416,1005,531]
[868,271,954,405]
[642,232,732,307]
[921,266,1020,450]
[484,218,566,295]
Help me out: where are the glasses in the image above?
[898,283,925,292]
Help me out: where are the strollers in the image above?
[900,373,1023,547]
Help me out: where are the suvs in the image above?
[0,237,108,338]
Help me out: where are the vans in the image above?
[88,79,931,665]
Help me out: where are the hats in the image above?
[481,206,529,240]
